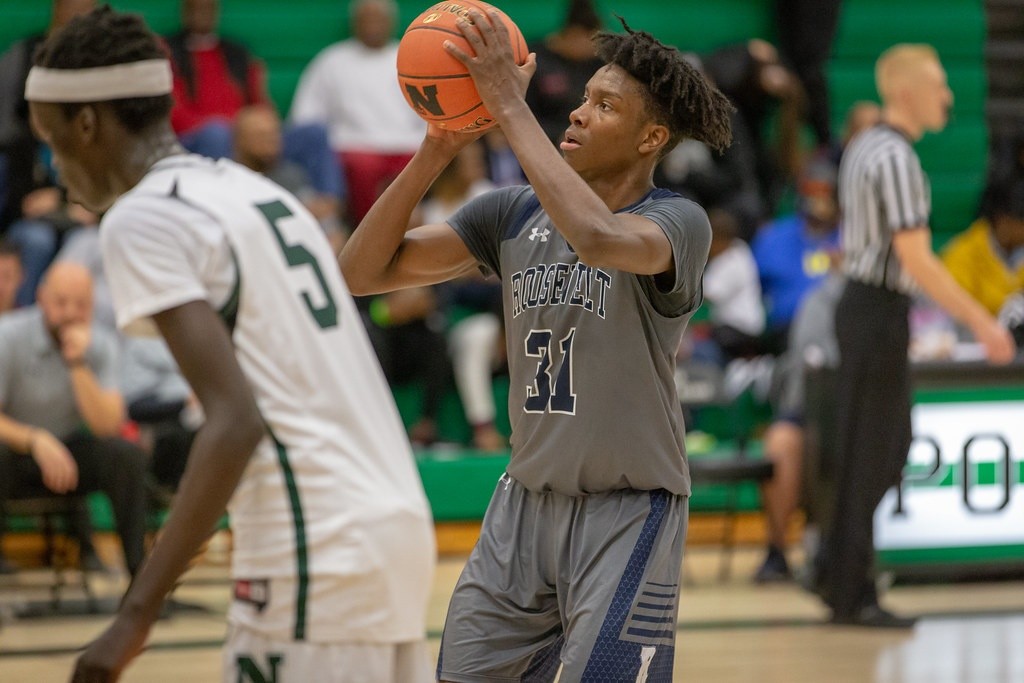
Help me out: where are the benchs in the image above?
[0,0,996,563]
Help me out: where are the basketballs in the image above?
[396,0,527,132]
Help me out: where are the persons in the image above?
[336,6,740,683]
[0,0,1024,634]
[24,3,437,683]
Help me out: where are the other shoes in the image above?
[755,558,790,584]
[826,596,921,628]
